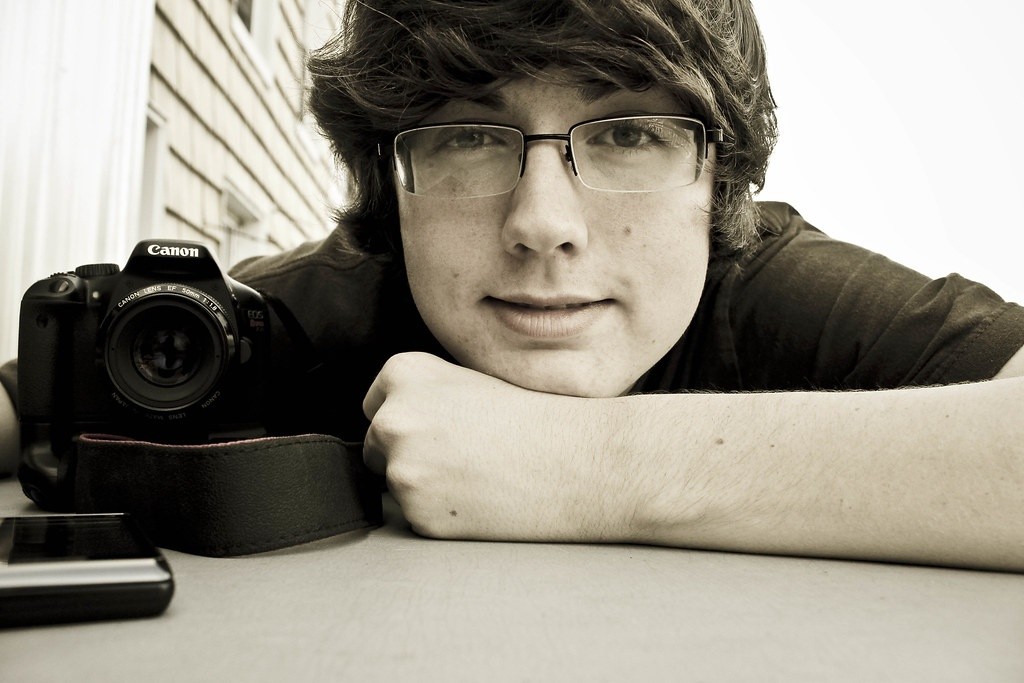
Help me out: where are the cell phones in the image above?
[0,515,173,631]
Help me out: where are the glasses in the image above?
[378,111,724,200]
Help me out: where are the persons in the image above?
[2,0,1024,569]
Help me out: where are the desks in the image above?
[0,489,1024,682]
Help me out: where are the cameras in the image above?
[17,240,287,513]
